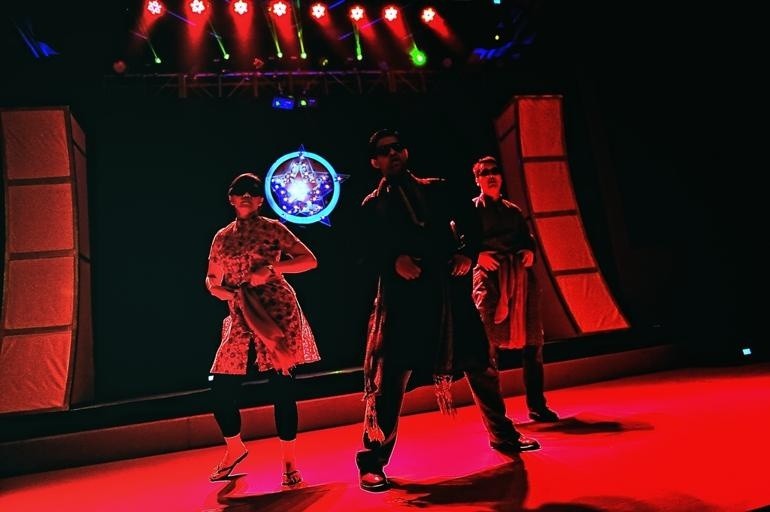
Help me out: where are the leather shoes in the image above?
[360,469,388,491]
[490,433,540,450]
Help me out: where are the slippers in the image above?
[282,470,301,485]
[209,452,248,481]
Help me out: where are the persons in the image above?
[205,173,321,486]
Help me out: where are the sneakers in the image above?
[529,407,559,422]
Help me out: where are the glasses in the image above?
[229,185,263,197]
[375,142,404,156]
[479,167,500,177]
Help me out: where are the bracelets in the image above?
[264,263,276,276]
